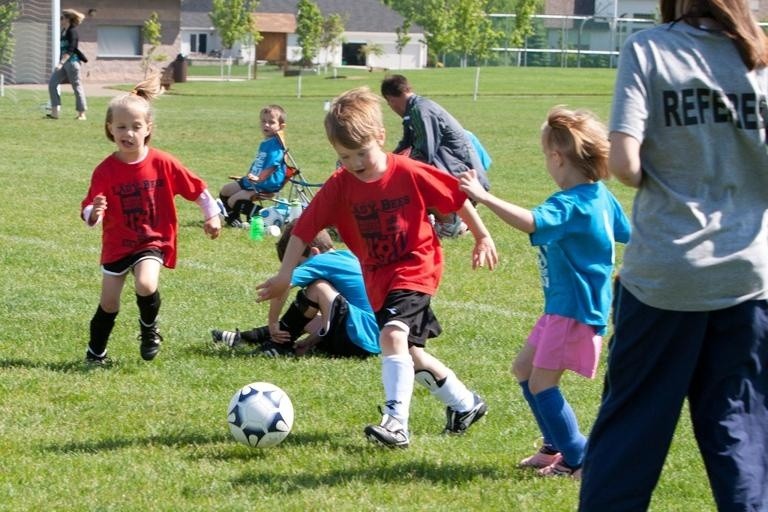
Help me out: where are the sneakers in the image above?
[211,327,241,349]
[256,342,294,357]
[365,405,408,452]
[434,216,467,240]
[441,394,488,436]
[519,444,563,469]
[536,454,582,479]
[138,319,163,361]
[88,349,107,361]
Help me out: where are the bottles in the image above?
[261,206,284,229]
[278,199,288,218]
[290,202,303,221]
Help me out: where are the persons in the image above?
[43,8,89,123]
[463,129,494,172]
[78,61,222,368]
[575,0,768,512]
[454,99,635,479]
[208,216,385,360]
[215,103,287,228]
[377,72,488,236]
[255,83,500,451]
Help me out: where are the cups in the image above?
[250,216,264,241]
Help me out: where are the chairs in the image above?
[227,164,300,227]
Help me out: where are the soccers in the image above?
[256,207,285,232]
[226,382,294,447]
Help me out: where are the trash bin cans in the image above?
[174,53,188,83]
